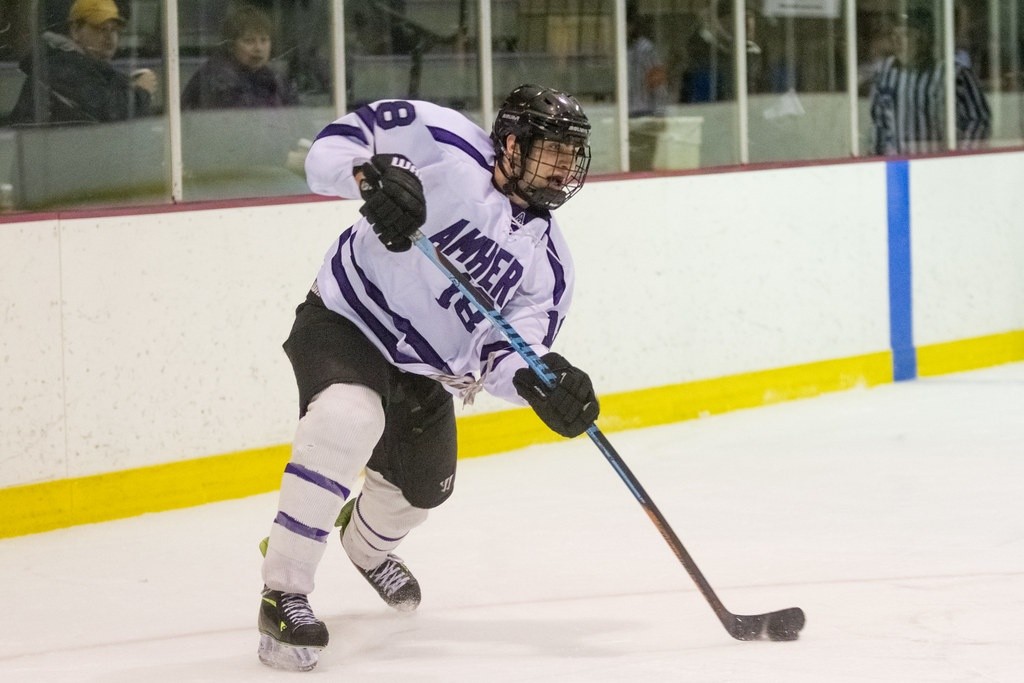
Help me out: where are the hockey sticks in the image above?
[350,156,806,643]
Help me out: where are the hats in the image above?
[70,0,123,25]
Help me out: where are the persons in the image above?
[256,84,600,673]
[868,0,992,155]
[626,0,789,116]
[4,0,301,125]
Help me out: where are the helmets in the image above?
[887,5,934,63]
[490,83,592,209]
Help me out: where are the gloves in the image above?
[513,352,599,437]
[361,152,427,251]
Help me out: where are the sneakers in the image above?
[338,498,421,613]
[258,536,329,670]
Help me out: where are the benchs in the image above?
[0,0,636,213]
